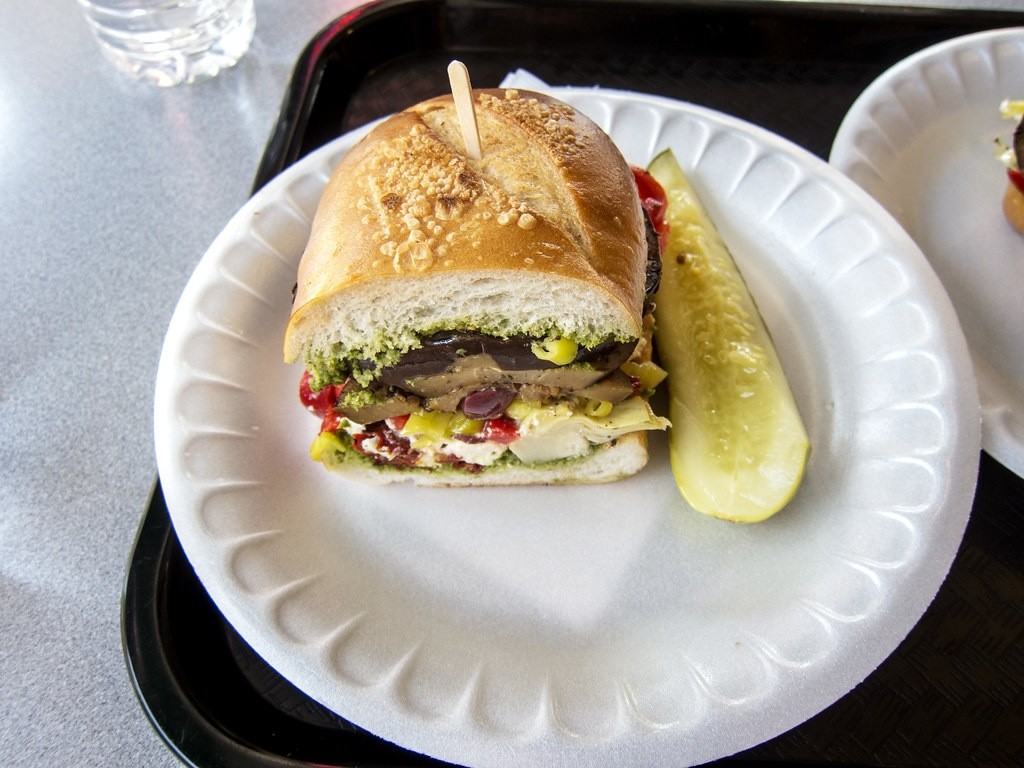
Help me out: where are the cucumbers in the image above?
[642,136,810,527]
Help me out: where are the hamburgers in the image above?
[284,91,671,491]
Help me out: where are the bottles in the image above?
[78,0,256,87]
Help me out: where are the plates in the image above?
[828,29,1024,484]
[148,91,982,768]
